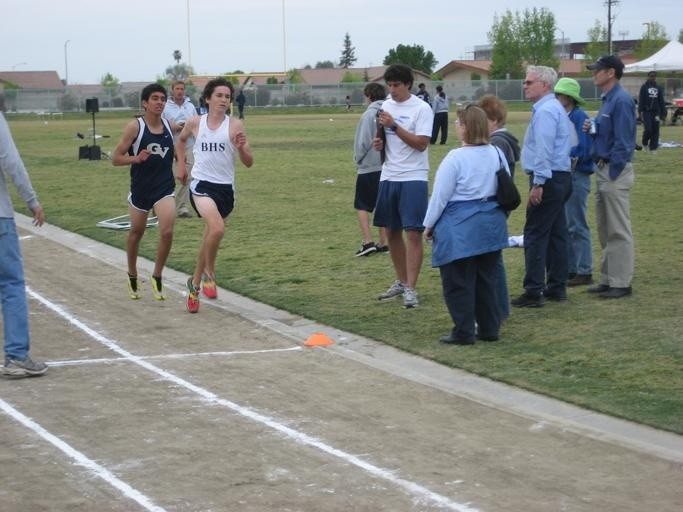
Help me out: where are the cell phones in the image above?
[376,108,385,118]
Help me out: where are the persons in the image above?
[477,94,521,175]
[513,64,573,308]
[638,71,666,154]
[553,76,596,287]
[354,83,390,257]
[422,104,509,346]
[417,82,448,144]
[0,111,50,379]
[583,54,636,299]
[111,77,252,313]
[375,65,434,309]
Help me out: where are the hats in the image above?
[554,78,588,106]
[586,55,624,71]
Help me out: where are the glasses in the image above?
[523,80,536,86]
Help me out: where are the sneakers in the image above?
[2,357,47,376]
[403,287,418,309]
[377,280,405,301]
[355,241,377,257]
[127,273,142,299]
[203,270,217,299]
[440,273,632,345]
[375,243,390,254]
[186,278,201,313]
[150,274,166,301]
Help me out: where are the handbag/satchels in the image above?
[496,168,521,213]
